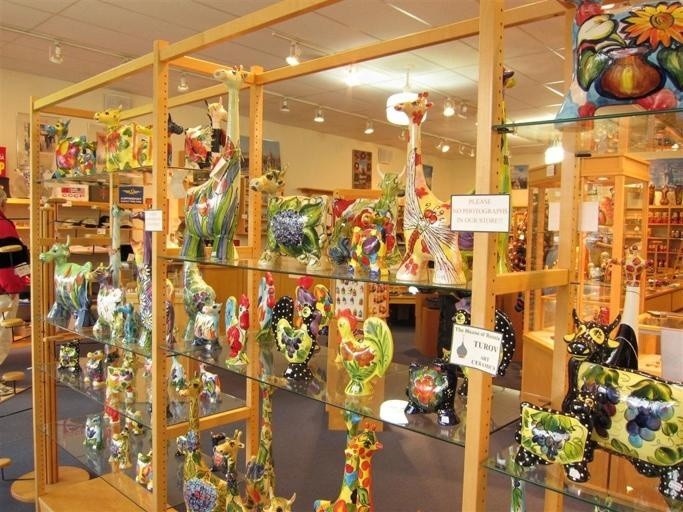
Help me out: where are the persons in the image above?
[0,184,33,397]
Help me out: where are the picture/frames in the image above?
[15,109,66,172]
[103,93,133,125]
[186,134,283,196]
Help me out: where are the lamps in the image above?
[265,30,527,140]
[44,42,478,159]
[385,64,427,126]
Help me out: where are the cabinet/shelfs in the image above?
[3,196,148,308]
[26,39,258,512]
[480,2,683,511]
[151,0,479,512]
